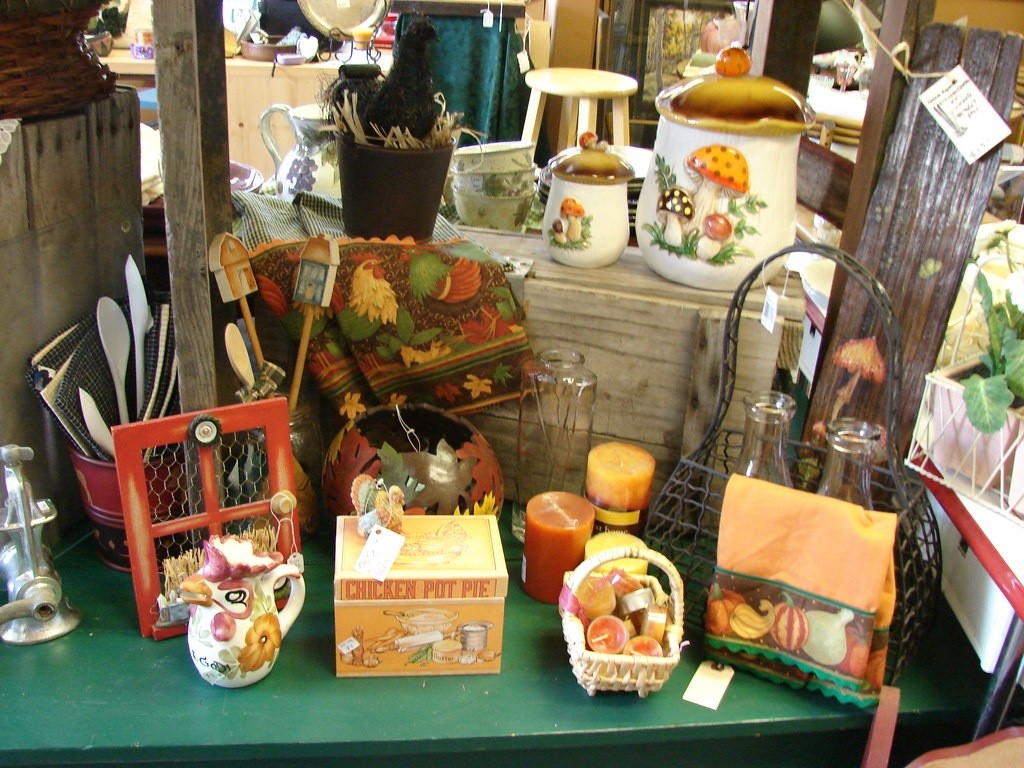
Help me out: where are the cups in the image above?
[809,74,834,103]
[135,27,152,47]
[634,45,819,291]
[335,137,452,246]
[536,131,636,269]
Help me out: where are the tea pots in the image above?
[180,536,305,690]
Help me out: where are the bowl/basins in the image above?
[239,35,297,62]
[86,32,112,58]
[131,44,155,59]
[452,139,539,236]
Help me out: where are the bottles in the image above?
[738,390,795,487]
[510,343,600,544]
[813,419,882,512]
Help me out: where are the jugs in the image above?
[255,104,340,201]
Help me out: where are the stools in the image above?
[523,66,637,163]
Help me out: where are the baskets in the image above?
[558,548,687,699]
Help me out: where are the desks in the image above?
[99,48,394,185]
[1,500,972,768]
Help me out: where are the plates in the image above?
[808,92,867,148]
[541,145,654,235]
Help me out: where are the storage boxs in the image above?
[454,222,809,526]
